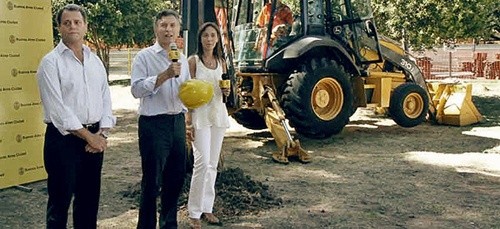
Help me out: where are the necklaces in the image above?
[206,62,214,66]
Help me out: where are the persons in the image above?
[257,0,294,62]
[36,4,115,229]
[186,22,232,229]
[130,8,192,229]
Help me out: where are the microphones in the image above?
[222,73,229,103]
[170,42,180,77]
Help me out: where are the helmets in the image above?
[178,79,213,109]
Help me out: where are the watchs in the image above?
[99,131,108,138]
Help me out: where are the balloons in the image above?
[178,80,214,109]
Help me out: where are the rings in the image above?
[226,93,229,95]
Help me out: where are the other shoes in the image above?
[201,213,223,226]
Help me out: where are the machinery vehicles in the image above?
[179,0,488,164]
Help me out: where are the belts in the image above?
[83,122,101,133]
